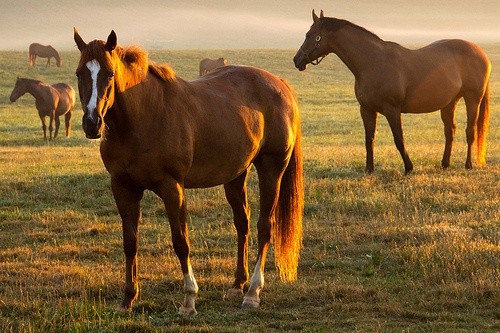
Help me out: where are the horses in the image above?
[28,43,62,69]
[9,75,76,140]
[293,8,491,176]
[73,26,305,321]
